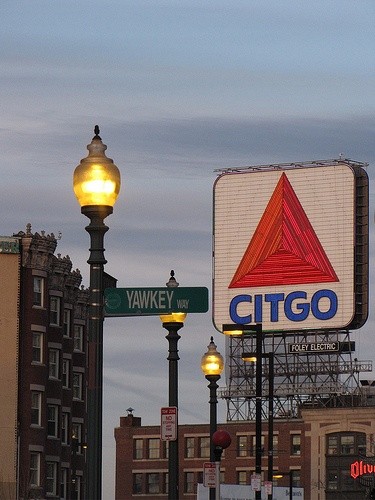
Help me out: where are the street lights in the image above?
[241,352,273,500]
[273,470,293,500]
[221,323,262,499]
[159,269,187,500]
[71,125,122,500]
[198,335,224,500]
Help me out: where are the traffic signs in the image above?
[102,283,209,318]
